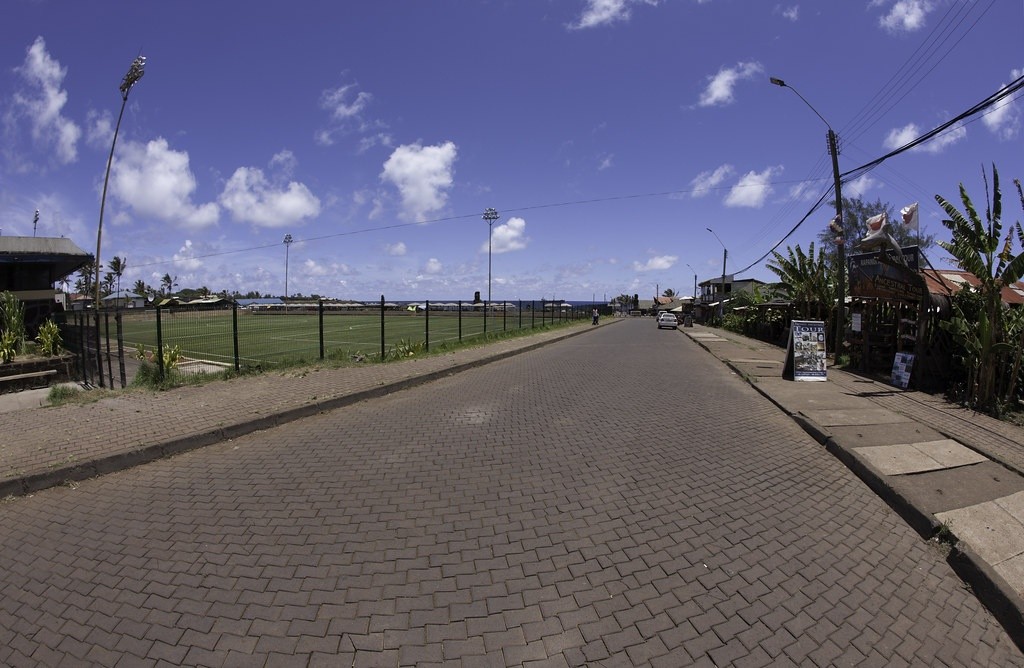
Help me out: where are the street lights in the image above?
[769,74,847,366]
[686,263,698,316]
[481,207,500,317]
[282,234,293,315]
[93,52,151,314]
[705,226,727,328]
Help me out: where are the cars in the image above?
[656,313,679,330]
[655,310,668,322]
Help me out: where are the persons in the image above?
[592,307,598,324]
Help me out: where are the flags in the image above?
[862,212,888,241]
[900,203,917,232]
[829,215,843,245]
